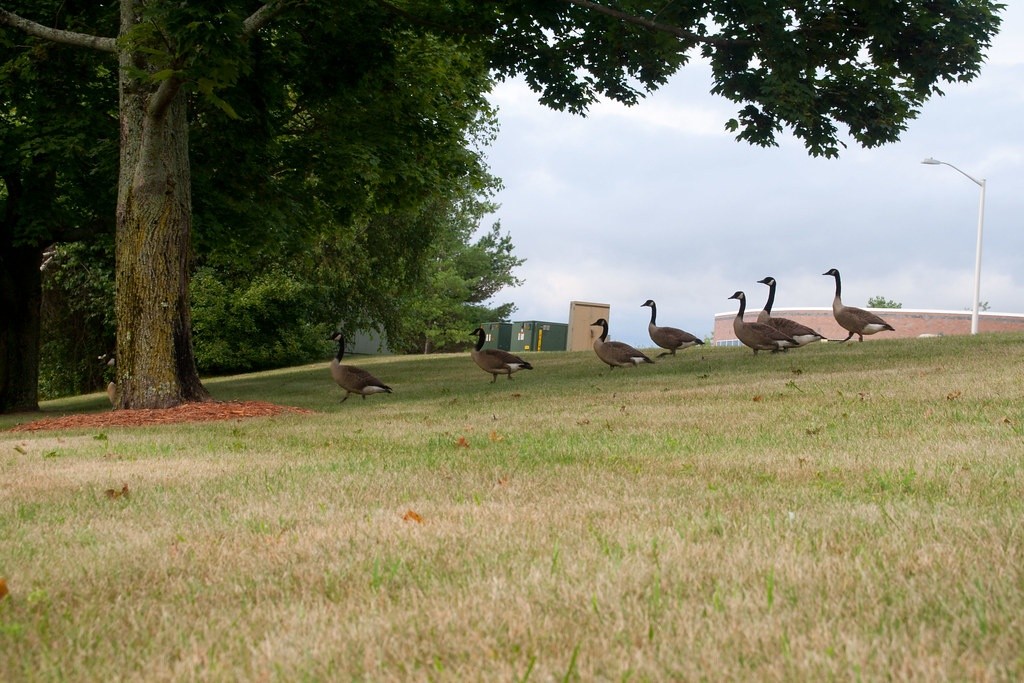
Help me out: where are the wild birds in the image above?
[728,276,828,355]
[640,299,706,358]
[325,332,393,404]
[590,318,656,371]
[822,268,896,344]
[97,349,117,406]
[468,327,534,384]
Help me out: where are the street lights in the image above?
[920,157,988,335]
